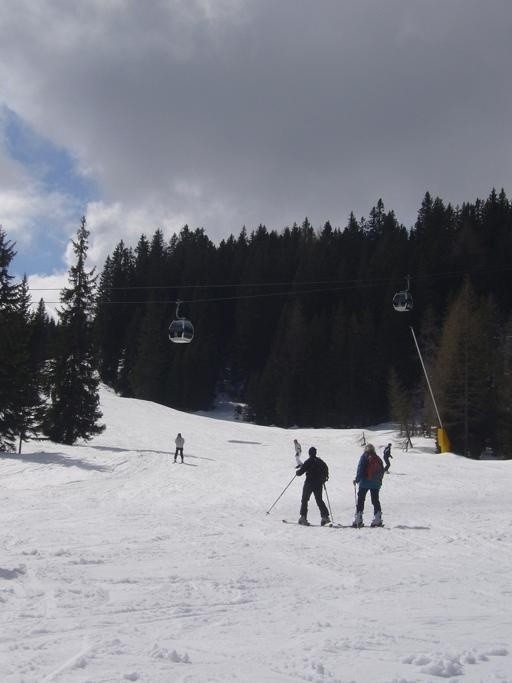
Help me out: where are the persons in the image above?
[294,439,331,525]
[174,433,184,463]
[352,443,393,527]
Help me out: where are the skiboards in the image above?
[345,519,390,529]
[283,521,341,527]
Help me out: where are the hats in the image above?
[309,447,317,456]
[366,444,374,453]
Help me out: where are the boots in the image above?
[172,459,184,464]
[352,511,364,527]
[371,511,383,527]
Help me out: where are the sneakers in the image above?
[321,516,331,526]
[296,464,303,469]
[298,513,308,524]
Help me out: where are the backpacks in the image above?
[310,458,329,486]
